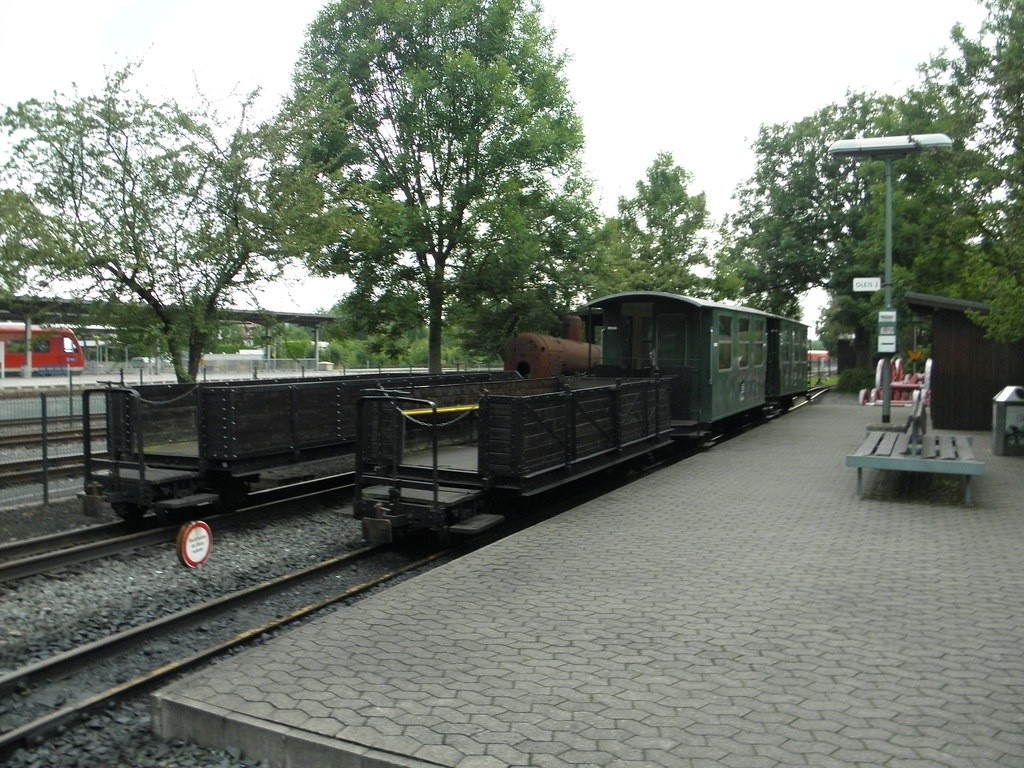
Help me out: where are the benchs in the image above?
[847,390,985,508]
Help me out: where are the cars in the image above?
[132,357,173,367]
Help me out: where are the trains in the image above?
[807,350,832,371]
[0,323,88,376]
[328,290,811,547]
[50,324,121,350]
[77,363,503,528]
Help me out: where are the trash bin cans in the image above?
[992,385,1024,456]
